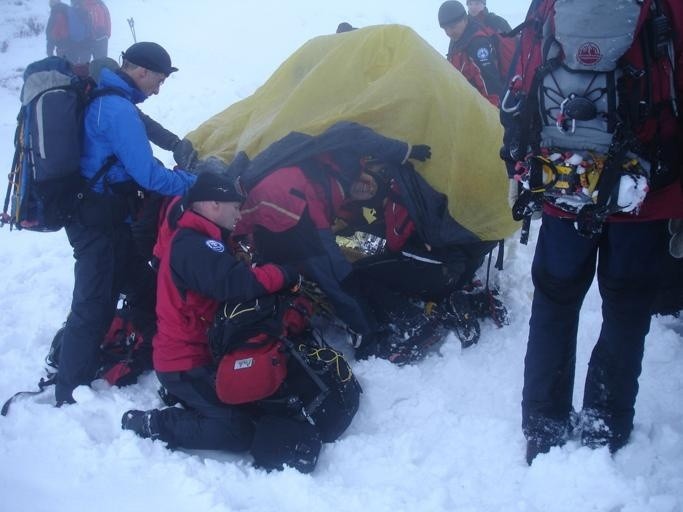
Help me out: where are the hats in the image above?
[186,170,246,203]
[439,0,466,28]
[121,41,178,76]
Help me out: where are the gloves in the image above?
[509,176,542,222]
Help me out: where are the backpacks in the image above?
[210,296,362,442]
[43,292,153,391]
[10,58,84,234]
[500,1,683,245]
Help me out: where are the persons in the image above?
[224,121,431,364]
[71,0,109,62]
[333,160,508,367]
[121,166,302,452]
[465,1,513,39]
[500,0,682,467]
[45,0,89,64]
[438,1,501,110]
[45,42,226,407]
